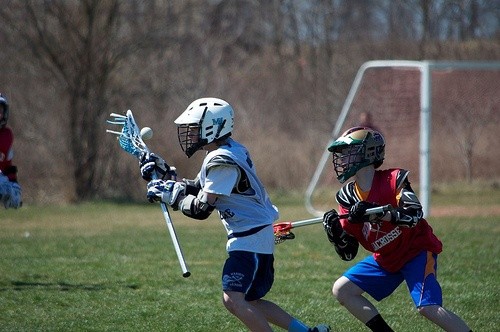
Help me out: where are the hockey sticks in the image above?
[118,109,191,278]
[272,204,392,246]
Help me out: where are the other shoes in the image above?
[312,324,331,332]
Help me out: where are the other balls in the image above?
[140,126,153,141]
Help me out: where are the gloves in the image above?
[321,209,346,246]
[139,152,177,182]
[4,181,23,211]
[349,201,387,224]
[146,179,186,212]
[0,175,12,202]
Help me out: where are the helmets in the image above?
[0,95,9,128]
[174,97,235,158]
[328,125,386,185]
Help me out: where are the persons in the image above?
[136,97,334,332]
[321,124,476,332]
[0,96,24,210]
